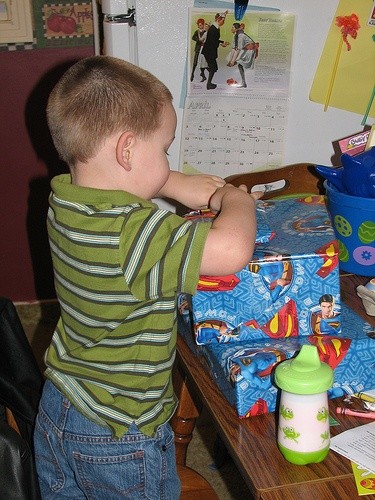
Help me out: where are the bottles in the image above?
[274,345,333,466]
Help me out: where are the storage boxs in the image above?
[175,296,375,418]
[188,197,342,345]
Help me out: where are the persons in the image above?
[34,56,266,500]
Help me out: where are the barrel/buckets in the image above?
[324,164,375,277]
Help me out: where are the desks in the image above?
[260,475,375,500]
[175,272,375,500]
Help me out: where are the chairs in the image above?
[226,163,325,200]
[0,295,219,500]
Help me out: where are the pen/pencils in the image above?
[336,405,375,421]
[354,392,375,403]
